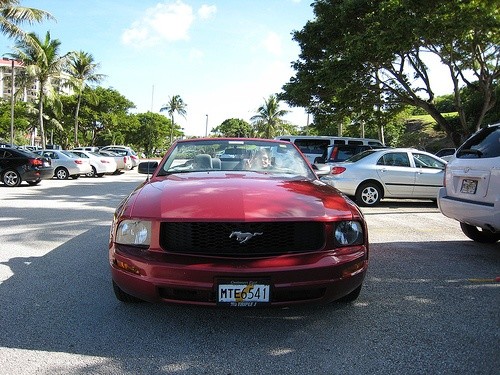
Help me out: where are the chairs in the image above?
[194,154,222,170]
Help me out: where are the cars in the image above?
[312,144,374,178]
[436,122,500,244]
[33,150,93,180]
[71,145,140,175]
[0,148,54,187]
[321,148,448,207]
[67,150,117,178]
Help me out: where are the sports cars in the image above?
[108,136,372,312]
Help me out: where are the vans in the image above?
[271,136,384,185]
